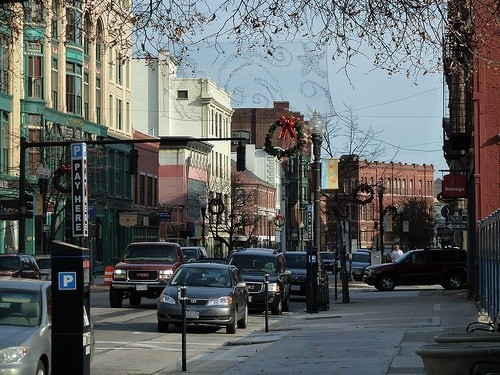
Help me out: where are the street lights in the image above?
[307,109,326,316]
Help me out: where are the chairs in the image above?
[17,302,50,327]
[264,262,275,271]
[217,275,229,286]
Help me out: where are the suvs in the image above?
[223,247,292,316]
[109,241,183,309]
[181,245,205,264]
[283,250,308,297]
[0,254,43,284]
[361,248,471,289]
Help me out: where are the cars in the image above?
[156,262,249,334]
[0,277,96,375]
[350,251,373,280]
[34,255,51,278]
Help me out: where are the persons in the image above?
[390,244,403,262]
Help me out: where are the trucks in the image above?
[320,250,338,272]
